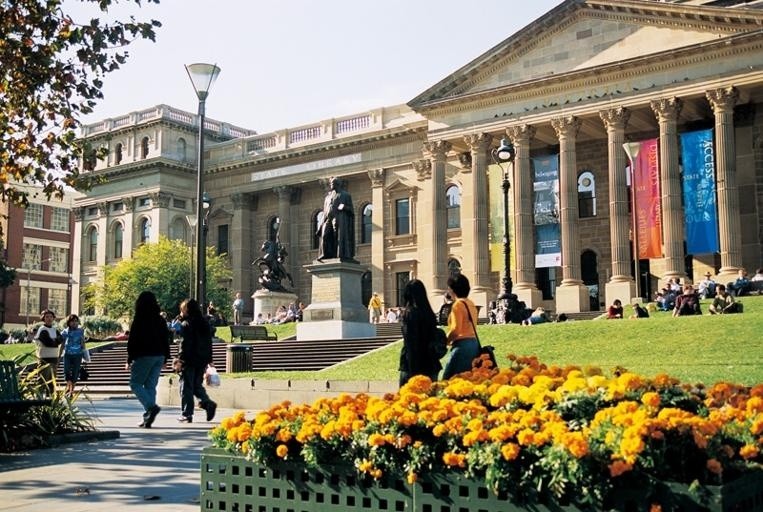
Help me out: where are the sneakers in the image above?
[140,406,160,428]
[207,401,216,420]
[177,415,192,423]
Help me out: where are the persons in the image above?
[171,297,217,423]
[252,300,305,327]
[152,298,224,336]
[33,309,64,397]
[521,305,553,326]
[84,330,130,343]
[442,273,481,381]
[398,279,447,389]
[124,290,170,429]
[58,314,85,399]
[232,292,244,325]
[385,308,396,323]
[367,292,382,325]
[315,177,355,259]
[606,264,763,319]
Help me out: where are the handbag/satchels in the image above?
[80,368,88,381]
[433,328,447,359]
[477,346,497,368]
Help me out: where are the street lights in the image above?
[26,257,52,325]
[196,190,212,315]
[181,60,221,315]
[489,137,519,319]
[619,142,641,297]
[184,215,197,296]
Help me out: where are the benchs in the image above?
[0,359,54,451]
[436,303,485,327]
[229,325,278,342]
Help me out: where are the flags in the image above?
[487,161,517,271]
[678,128,721,256]
[531,152,562,267]
[621,137,664,260]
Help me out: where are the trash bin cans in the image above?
[225,342,254,372]
